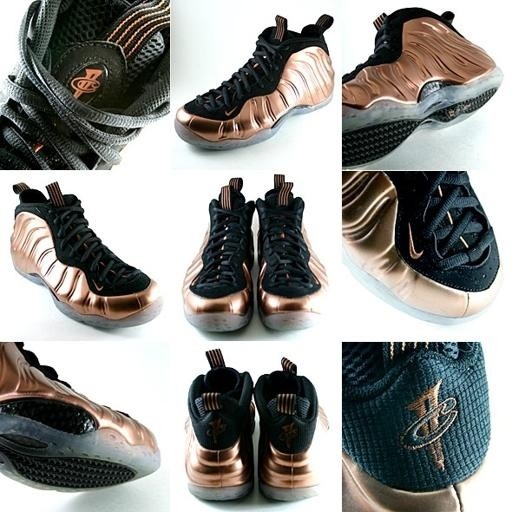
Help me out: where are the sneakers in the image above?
[342,171,500,318]
[254,358,329,501]
[256,175,329,331]
[183,178,255,332]
[0,0,170,170]
[342,8,503,167]
[186,349,255,501]
[0,342,160,488]
[342,342,490,512]
[175,14,334,150]
[11,183,162,328]
